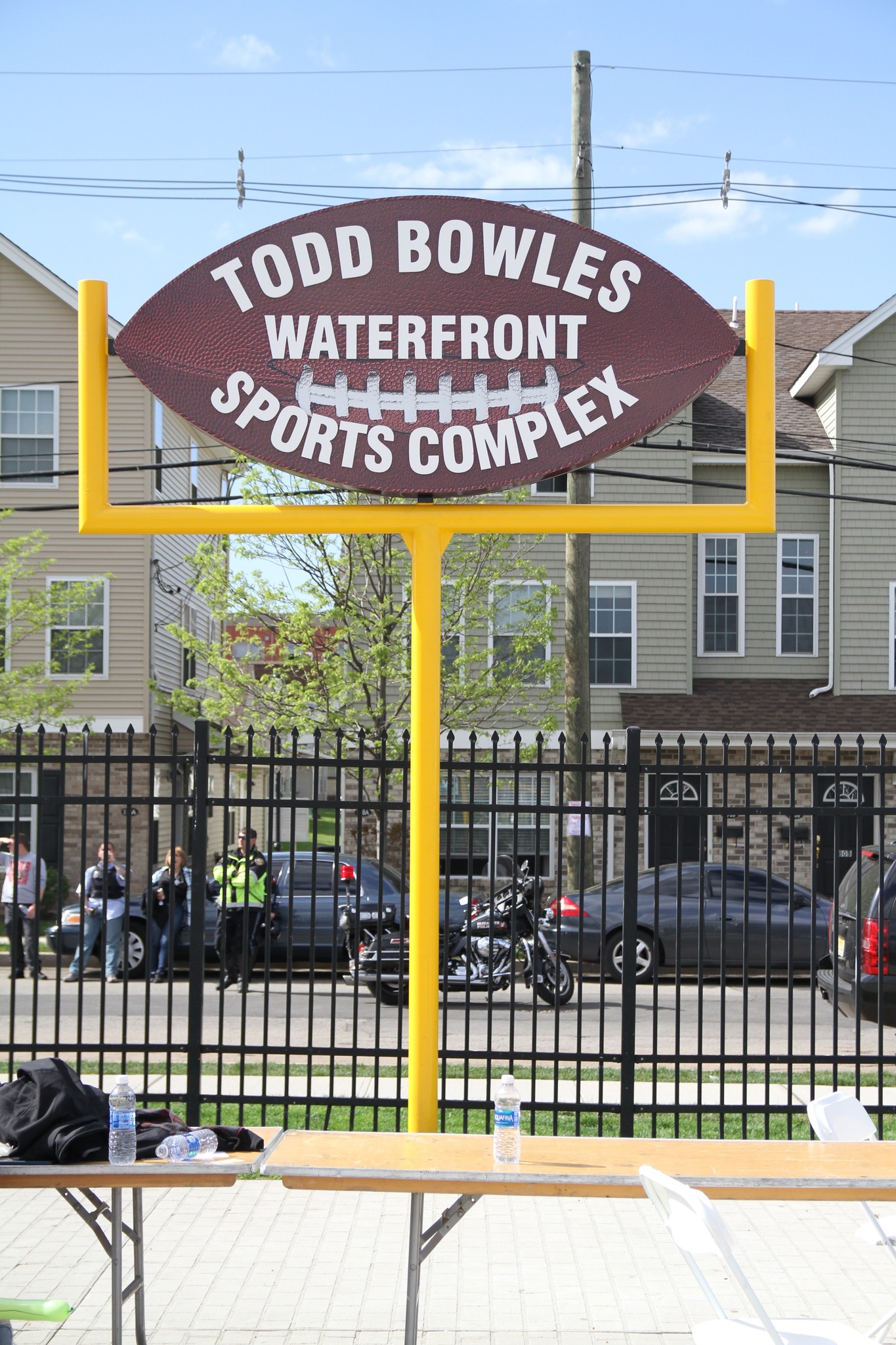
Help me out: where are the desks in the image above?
[0,1127,284,1345]
[260,1129,896,1345]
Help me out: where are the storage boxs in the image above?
[362,931,409,968]
[338,903,397,928]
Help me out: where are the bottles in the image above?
[18,904,28,915]
[108,1074,136,1165]
[155,1129,218,1162]
[494,1074,521,1164]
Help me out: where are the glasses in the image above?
[238,835,246,840]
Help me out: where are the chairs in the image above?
[640,1091,896,1345]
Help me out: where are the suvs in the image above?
[817,839,896,1031]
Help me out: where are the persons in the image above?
[150,846,192,983]
[0,833,47,980]
[63,841,133,983]
[212,828,267,993]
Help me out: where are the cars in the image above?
[43,849,489,981]
[537,861,833,986]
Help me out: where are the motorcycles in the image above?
[338,854,574,1007]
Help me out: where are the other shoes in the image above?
[216,974,237,991]
[31,971,47,979]
[153,973,165,983]
[8,967,25,979]
[237,977,250,993]
[62,974,79,982]
[107,974,118,983]
